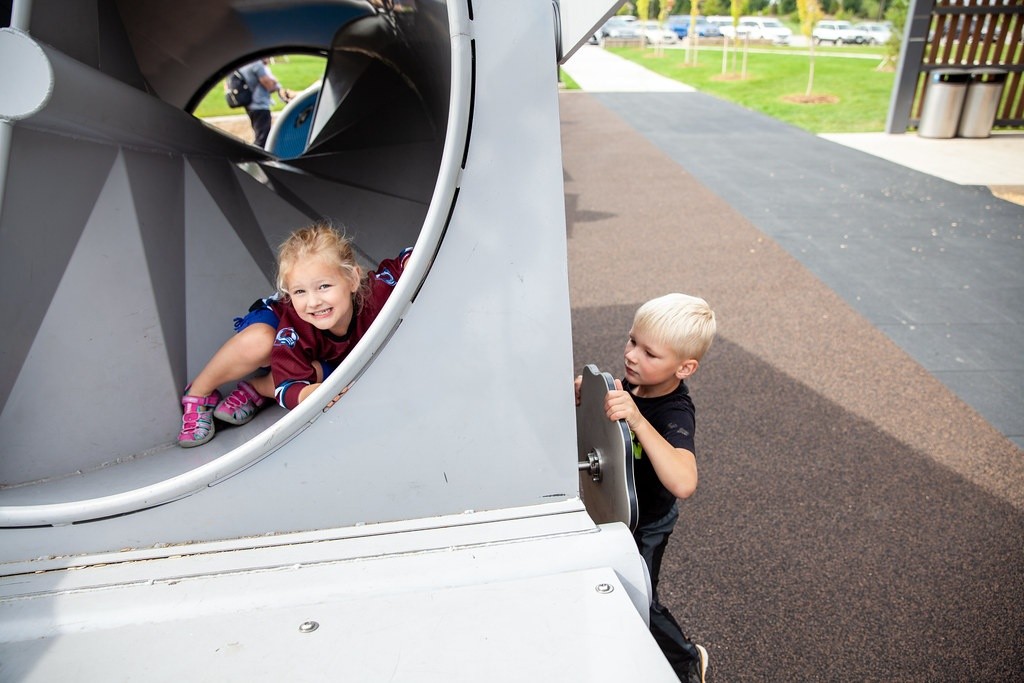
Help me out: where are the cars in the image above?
[600,12,794,47]
[812,19,866,46]
[940,14,1003,45]
[855,21,893,47]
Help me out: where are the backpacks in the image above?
[225,69,260,108]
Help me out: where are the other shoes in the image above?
[680,644,709,683]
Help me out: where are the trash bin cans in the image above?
[917,67,973,139]
[957,69,1010,138]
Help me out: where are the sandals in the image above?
[214,380,264,425]
[179,384,222,447]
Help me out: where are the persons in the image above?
[178,218,414,448]
[237,58,304,148]
[574,293,718,683]
